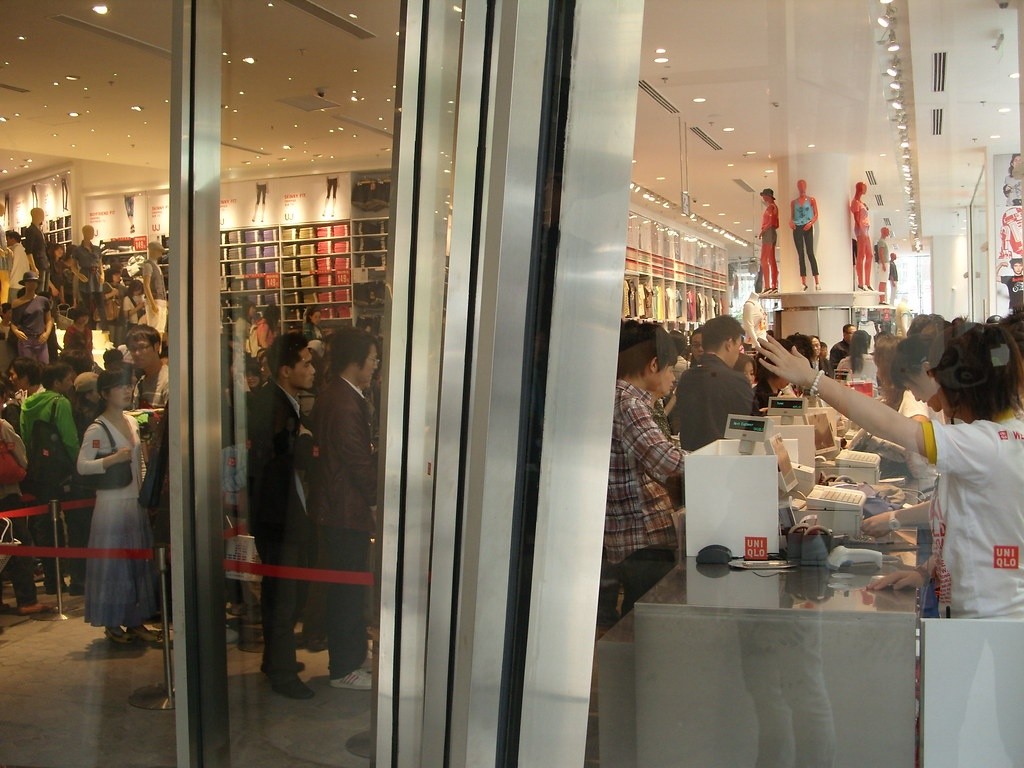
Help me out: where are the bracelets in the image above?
[914,565,930,579]
[810,370,825,399]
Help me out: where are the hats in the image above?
[760,188,776,200]
[18,271,40,287]
[74,372,100,392]
[306,340,325,358]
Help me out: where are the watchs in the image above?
[889,508,901,532]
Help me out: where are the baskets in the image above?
[225,535,262,583]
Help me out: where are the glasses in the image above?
[126,342,152,353]
[368,354,379,364]
[114,383,134,390]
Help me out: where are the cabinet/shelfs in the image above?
[161,218,390,345]
[619,246,730,347]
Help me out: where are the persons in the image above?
[744,291,767,348]
[676,315,754,453]
[759,189,779,295]
[790,179,820,293]
[9,272,53,368]
[756,324,1024,621]
[0,201,14,318]
[876,227,890,304]
[889,252,898,309]
[25,207,51,296]
[601,321,691,618]
[140,242,168,359]
[70,225,111,346]
[596,310,1024,636]
[851,182,874,292]
[0,233,379,698]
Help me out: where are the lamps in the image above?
[877,0,922,253]
[627,181,749,248]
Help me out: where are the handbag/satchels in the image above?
[71,419,133,489]
[93,282,118,320]
[24,395,76,499]
[128,295,146,321]
[0,420,26,483]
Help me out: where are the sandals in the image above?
[127,626,161,641]
[105,627,131,642]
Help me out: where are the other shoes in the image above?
[242,606,263,625]
[47,583,69,594]
[18,603,52,616]
[0,603,9,614]
[260,658,306,674]
[302,632,330,652]
[68,586,85,596]
[228,602,247,616]
[271,673,314,699]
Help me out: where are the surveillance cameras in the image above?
[315,86,327,98]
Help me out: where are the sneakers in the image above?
[359,649,373,672]
[329,668,372,689]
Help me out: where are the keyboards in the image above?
[806,485,868,510]
[835,449,881,468]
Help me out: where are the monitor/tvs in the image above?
[764,432,808,509]
[803,412,840,467]
